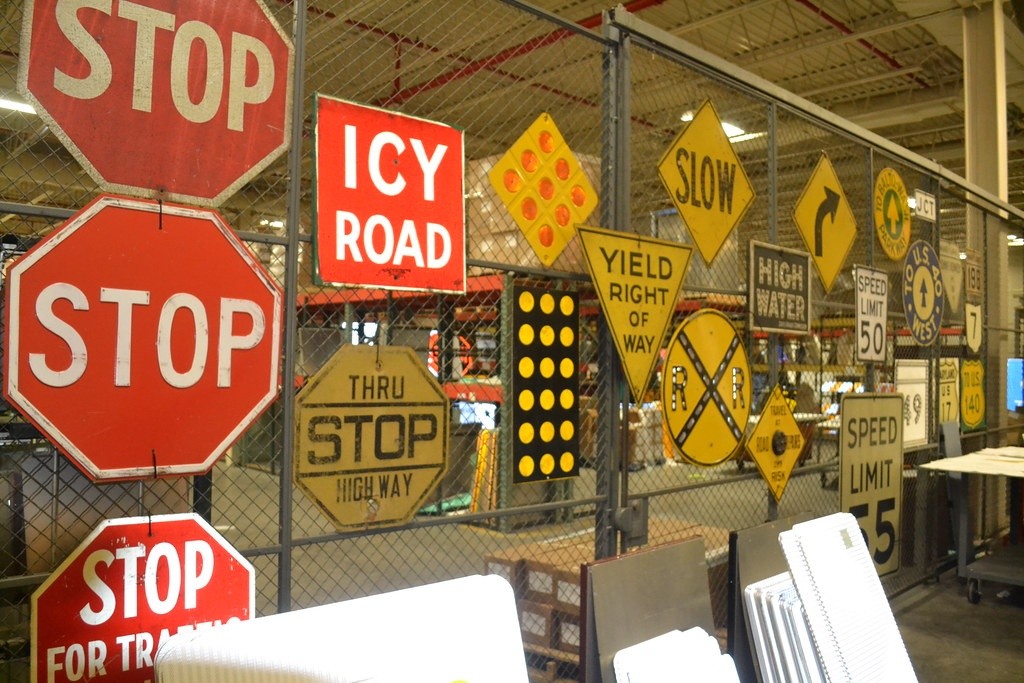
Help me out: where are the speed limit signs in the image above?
[851,262,891,363]
[836,387,906,581]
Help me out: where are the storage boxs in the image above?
[575,392,667,465]
[482,511,742,654]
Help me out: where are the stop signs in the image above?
[25,514,257,683]
[17,1,301,211]
[1,195,281,482]
[294,341,449,535]
